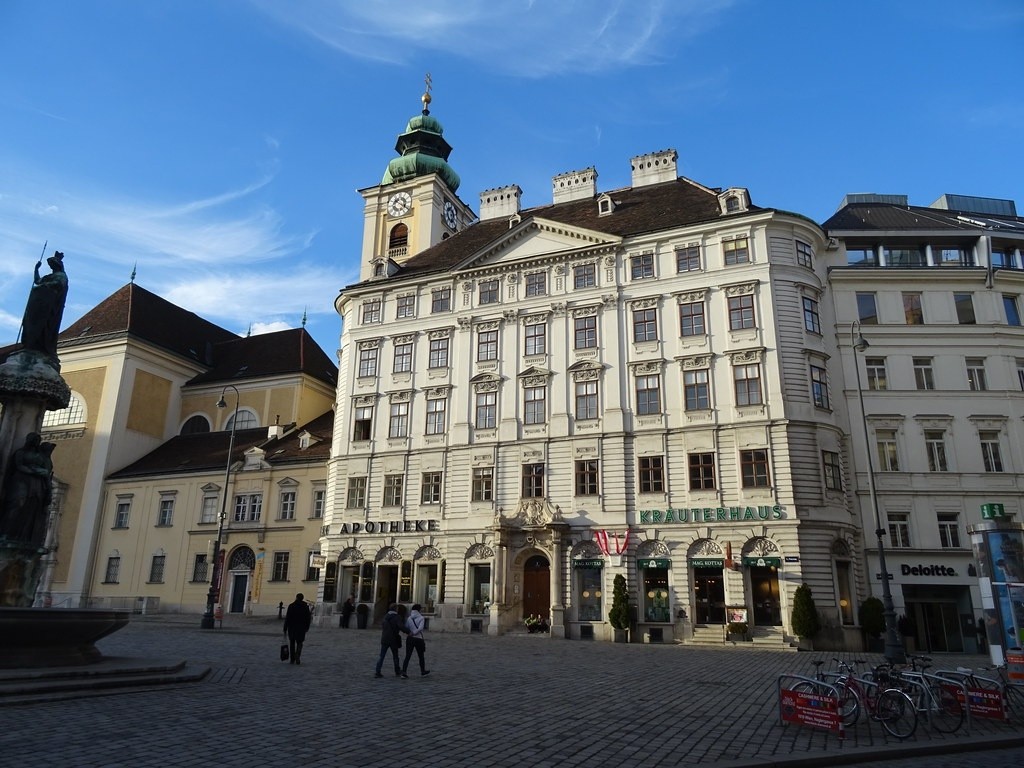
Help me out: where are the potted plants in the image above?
[609,573,630,642]
[792,583,819,652]
[897,614,916,653]
[727,622,748,641]
[979,617,990,656]
[858,595,887,653]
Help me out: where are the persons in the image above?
[524,613,549,634]
[0,433,58,546]
[341,598,354,629]
[21,251,68,361]
[400,603,431,678]
[283,593,312,664]
[374,603,415,679]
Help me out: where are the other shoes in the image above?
[295,655,300,665]
[374,673,385,678]
[528,631,531,633]
[544,631,546,634]
[400,674,409,679]
[290,657,294,664]
[538,631,541,633]
[421,670,431,678]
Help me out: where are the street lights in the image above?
[849,317,910,662]
[198,384,240,629]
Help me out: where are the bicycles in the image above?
[783,657,1024,739]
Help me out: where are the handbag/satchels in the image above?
[280,645,289,661]
[397,635,402,648]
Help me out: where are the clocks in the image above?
[388,192,411,217]
[444,202,458,228]
